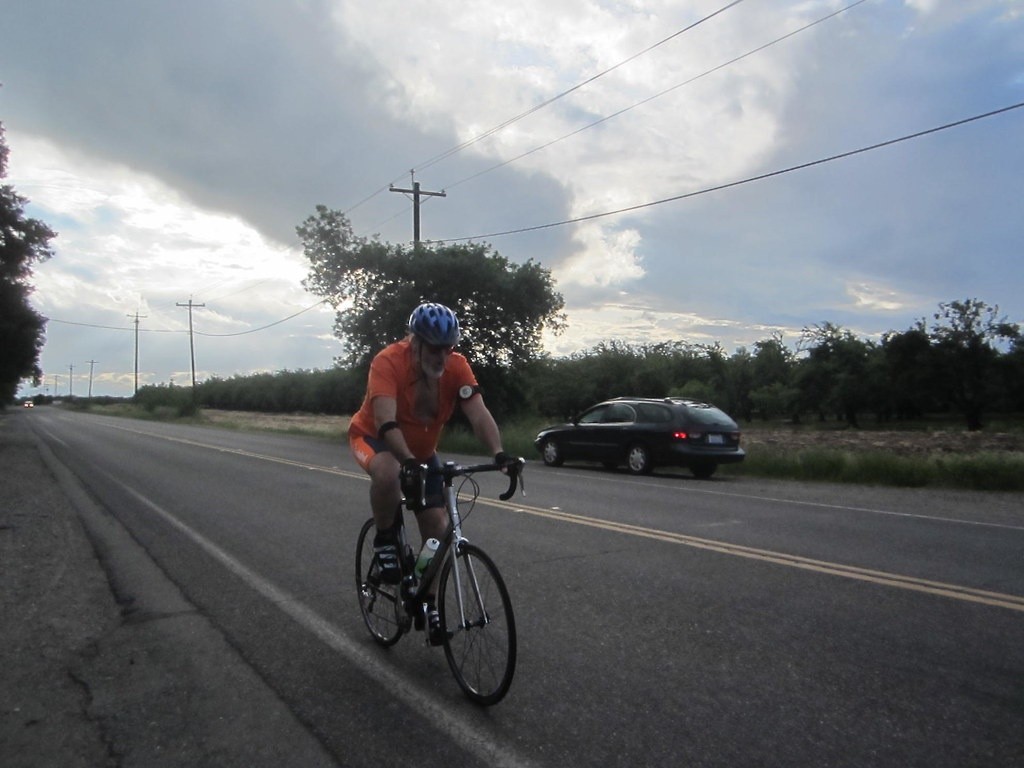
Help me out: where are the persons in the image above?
[347,302,522,645]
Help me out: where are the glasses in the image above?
[425,342,455,356]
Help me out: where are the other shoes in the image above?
[426,611,454,646]
[374,544,401,585]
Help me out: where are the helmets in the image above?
[409,303,461,345]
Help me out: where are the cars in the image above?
[24,400,34,408]
[535,395,745,476]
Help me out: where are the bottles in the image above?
[414,537,440,578]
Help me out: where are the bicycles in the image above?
[357,460,526,704]
